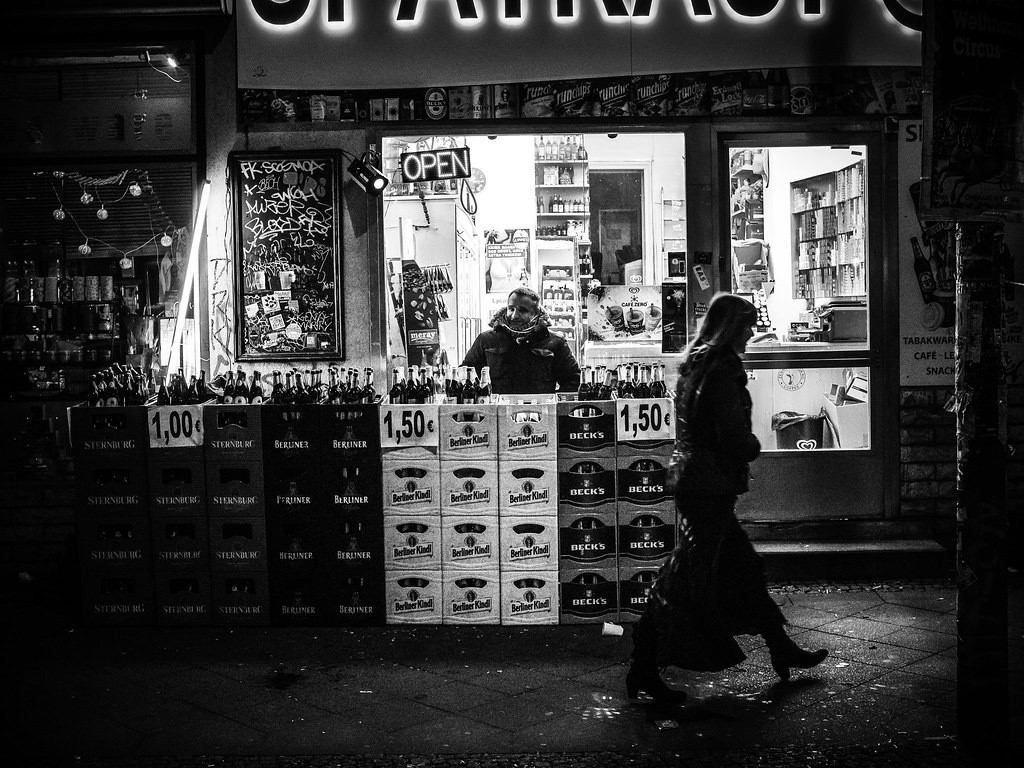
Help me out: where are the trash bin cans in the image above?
[772,412,825,450]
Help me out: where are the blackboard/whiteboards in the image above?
[228,149,345,361]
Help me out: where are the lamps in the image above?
[341,148,388,197]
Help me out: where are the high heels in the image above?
[626,663,687,704]
[769,642,828,679]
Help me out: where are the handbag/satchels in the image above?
[675,444,751,498]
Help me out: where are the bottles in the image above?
[536,133,594,341]
[2,272,668,595]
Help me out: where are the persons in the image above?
[457,287,583,394]
[624,293,831,704]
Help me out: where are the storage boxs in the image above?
[823,393,867,448]
[822,305,867,340]
[1,392,675,628]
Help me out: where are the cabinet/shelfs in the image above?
[790,159,867,298]
[729,148,765,296]
[535,159,591,342]
[2,299,126,391]
